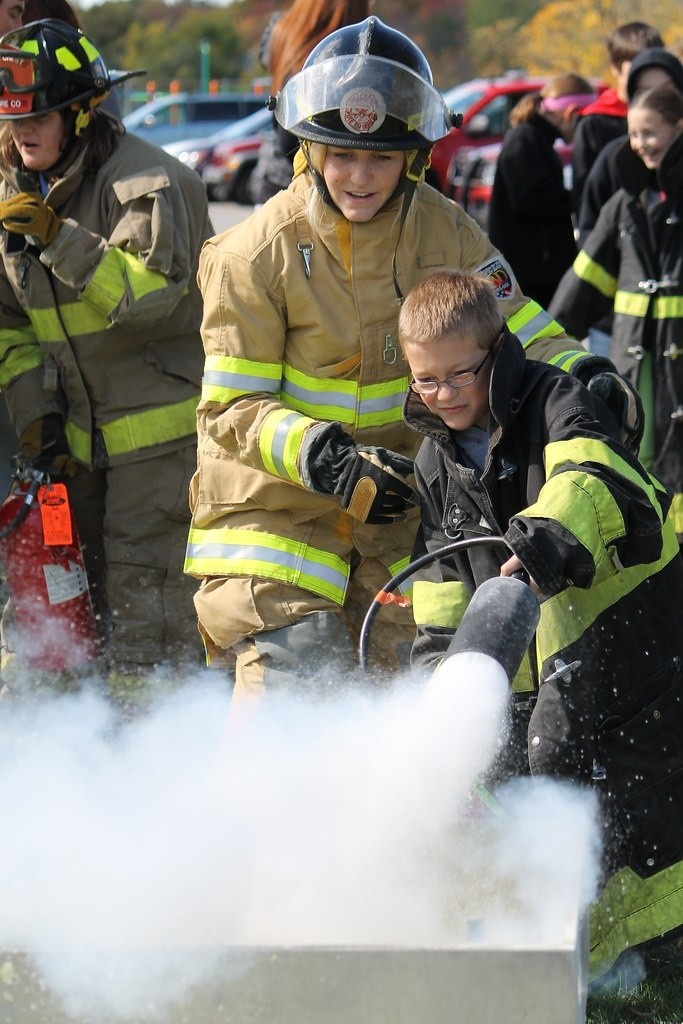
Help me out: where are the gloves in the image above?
[20,413,71,476]
[573,356,645,458]
[307,423,421,526]
[0,168,68,247]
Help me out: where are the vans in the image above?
[119,92,272,152]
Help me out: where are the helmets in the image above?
[0,18,149,120]
[265,15,463,150]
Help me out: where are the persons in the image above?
[182,12,646,704]
[1,1,125,150]
[393,269,683,1024]
[1,17,220,698]
[254,1,370,207]
[488,20,682,526]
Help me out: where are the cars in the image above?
[446,134,573,236]
[164,106,283,208]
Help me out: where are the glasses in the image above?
[408,346,494,395]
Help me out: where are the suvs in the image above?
[422,74,610,196]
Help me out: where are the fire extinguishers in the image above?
[359,535,540,708]
[0,437,100,671]
